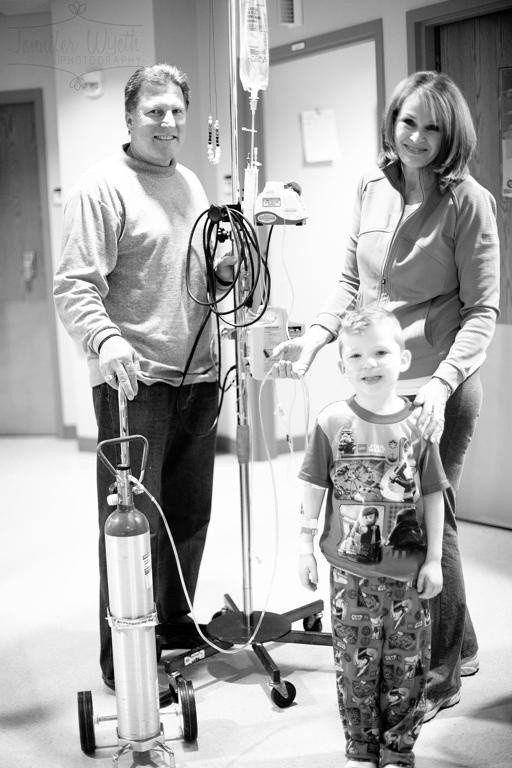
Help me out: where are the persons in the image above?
[349,507,382,564]
[267,70,501,723]
[298,304,450,768]
[53,63,251,691]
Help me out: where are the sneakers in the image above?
[423,652,480,722]
[156,624,234,648]
